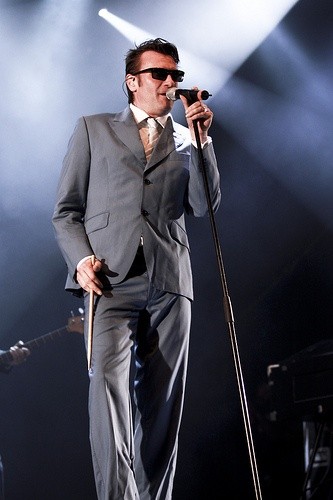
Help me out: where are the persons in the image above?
[52,38,223,500]
[0,340,31,371]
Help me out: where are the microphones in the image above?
[166,88,209,101]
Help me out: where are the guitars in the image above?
[0,307,86,365]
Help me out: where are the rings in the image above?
[202,106,207,112]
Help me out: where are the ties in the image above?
[145,118,160,163]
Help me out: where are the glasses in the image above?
[133,68,185,82]
